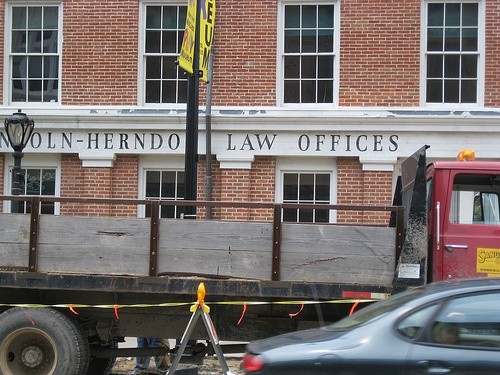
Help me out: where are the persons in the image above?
[132,337,172,375]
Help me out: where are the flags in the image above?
[177,0,197,74]
[199,0,216,84]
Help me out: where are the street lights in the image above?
[4,109,35,213]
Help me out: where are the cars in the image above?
[242,278,499,375]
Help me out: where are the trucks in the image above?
[2,147,500,374]
